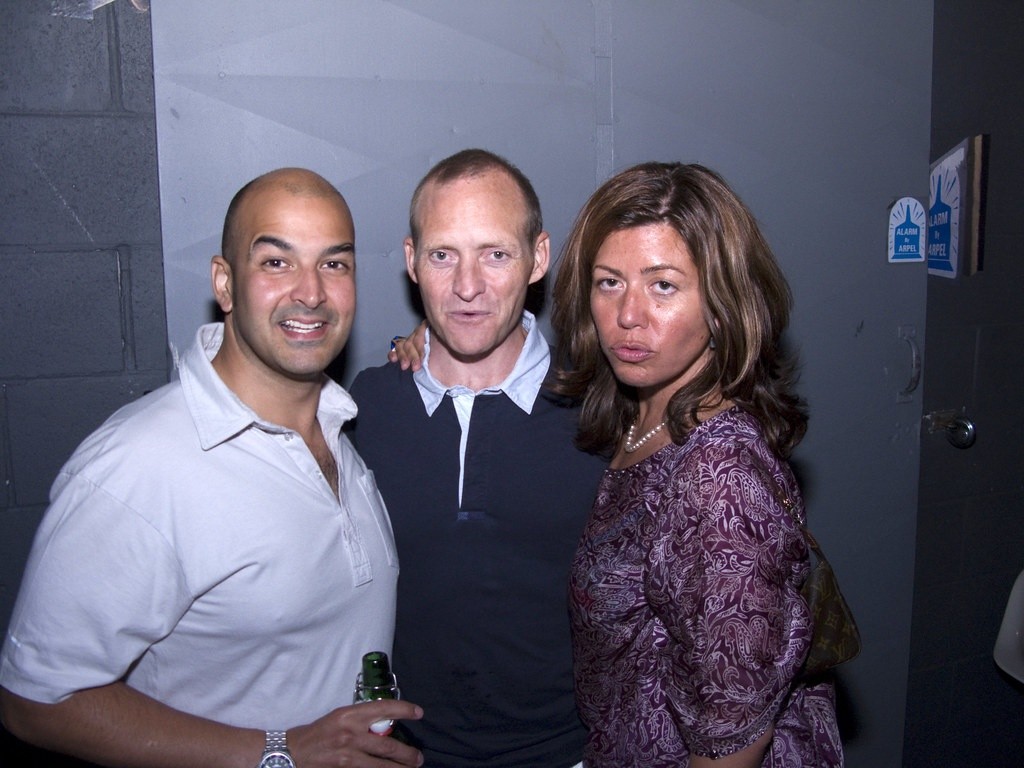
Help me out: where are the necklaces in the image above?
[626,419,667,452]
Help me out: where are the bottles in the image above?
[356,652,424,752]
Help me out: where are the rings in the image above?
[391,336,403,351]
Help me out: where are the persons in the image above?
[388,162,847,768]
[346,147,635,768]
[0,166,429,768]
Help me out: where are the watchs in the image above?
[258,729,296,768]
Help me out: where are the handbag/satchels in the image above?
[739,439,864,679]
